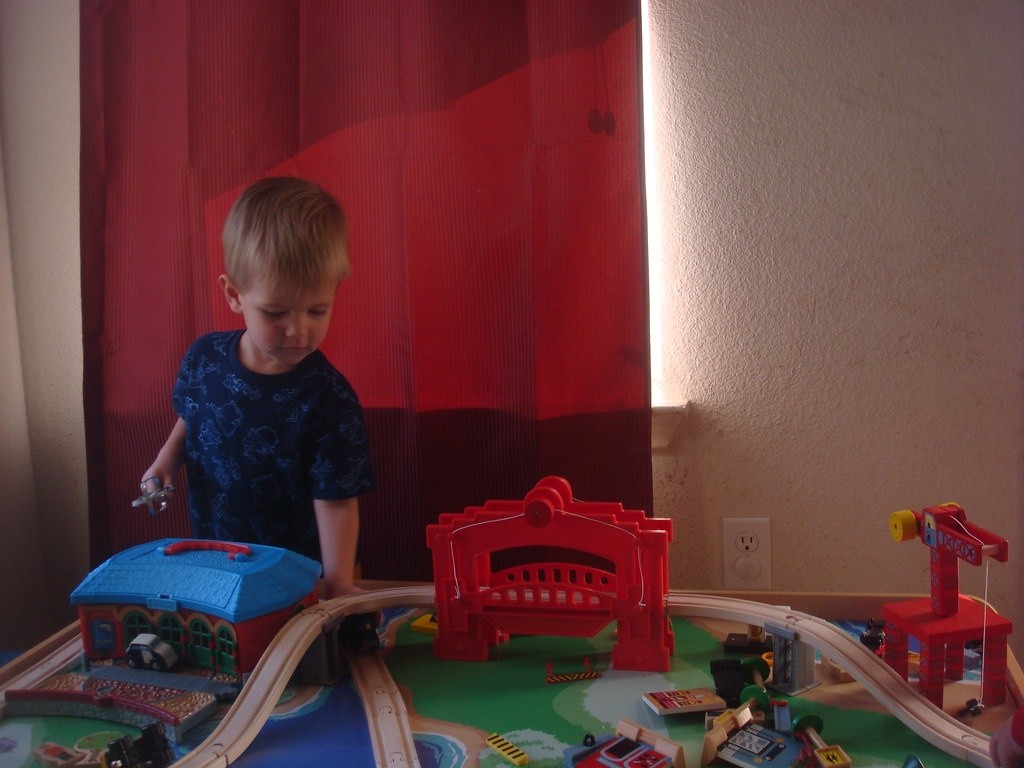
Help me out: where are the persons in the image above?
[141,175,382,631]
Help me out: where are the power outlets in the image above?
[724,517,772,590]
